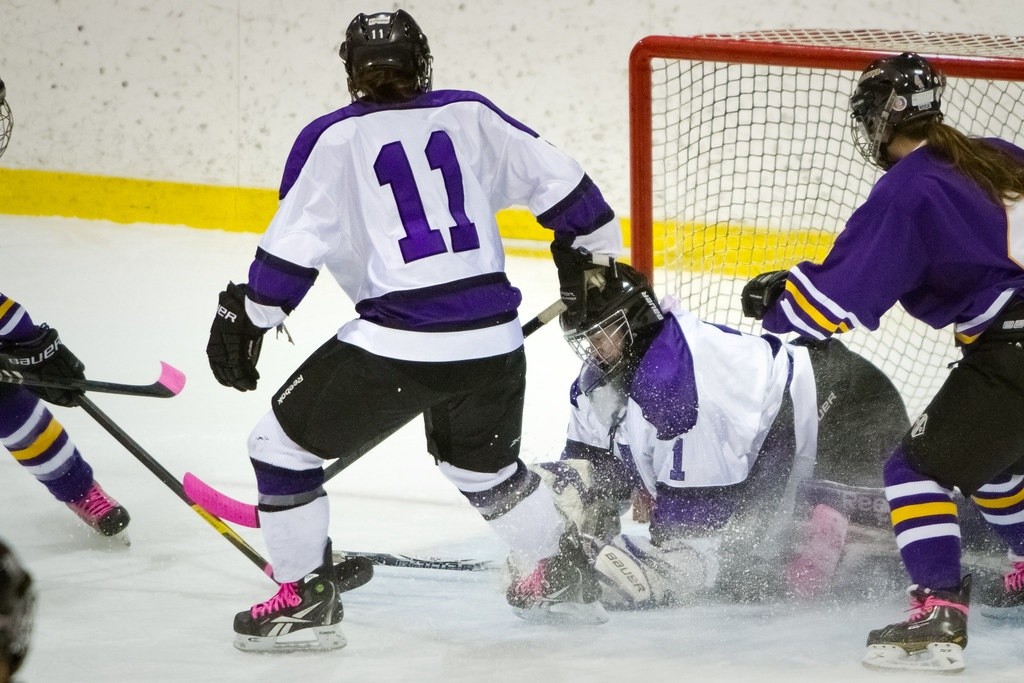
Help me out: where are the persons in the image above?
[740,49,1024,675]
[510,254,1009,625]
[207,6,624,656]
[0,79,130,537]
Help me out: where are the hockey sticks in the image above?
[0,357,188,400]
[66,378,376,599]
[183,289,571,530]
[332,548,503,574]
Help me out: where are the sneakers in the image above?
[863,573,973,674]
[63,477,131,547]
[979,547,1024,620]
[505,521,610,628]
[233,536,347,653]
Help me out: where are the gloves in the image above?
[551,242,616,329]
[0,323,85,407]
[206,281,272,392]
[742,270,792,319]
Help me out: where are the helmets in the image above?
[340,9,432,97]
[852,54,946,133]
[558,262,664,343]
